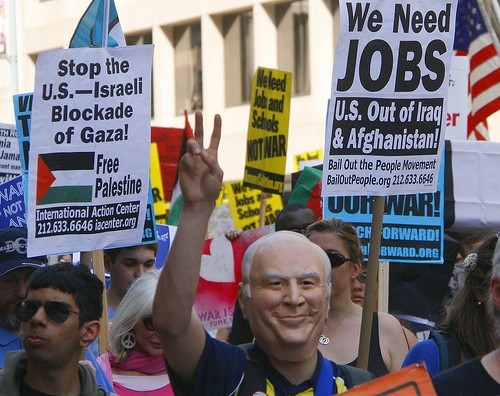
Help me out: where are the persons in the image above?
[153,110,373,396]
[1,203,498,396]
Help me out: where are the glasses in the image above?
[141,317,156,331]
[326,252,352,267]
[15,300,93,323]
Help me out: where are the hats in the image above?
[0,228,48,277]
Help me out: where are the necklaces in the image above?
[318,302,353,344]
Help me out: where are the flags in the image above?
[452,0,500,140]
